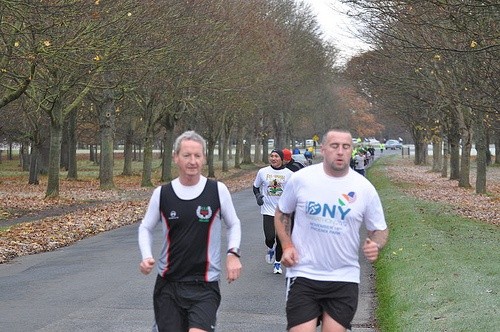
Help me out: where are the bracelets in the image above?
[228,247,241,257]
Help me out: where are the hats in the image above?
[293,148,300,154]
[282,149,292,160]
[271,149,284,160]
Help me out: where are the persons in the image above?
[274,126,389,332]
[138,130,243,332]
[253,141,386,274]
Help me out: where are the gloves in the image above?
[256,193,264,206]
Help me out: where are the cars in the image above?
[384,139,402,150]
[268,139,274,146]
[357,137,370,142]
[305,140,317,145]
[353,139,358,143]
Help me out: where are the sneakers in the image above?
[273,260,282,274]
[266,246,276,264]
